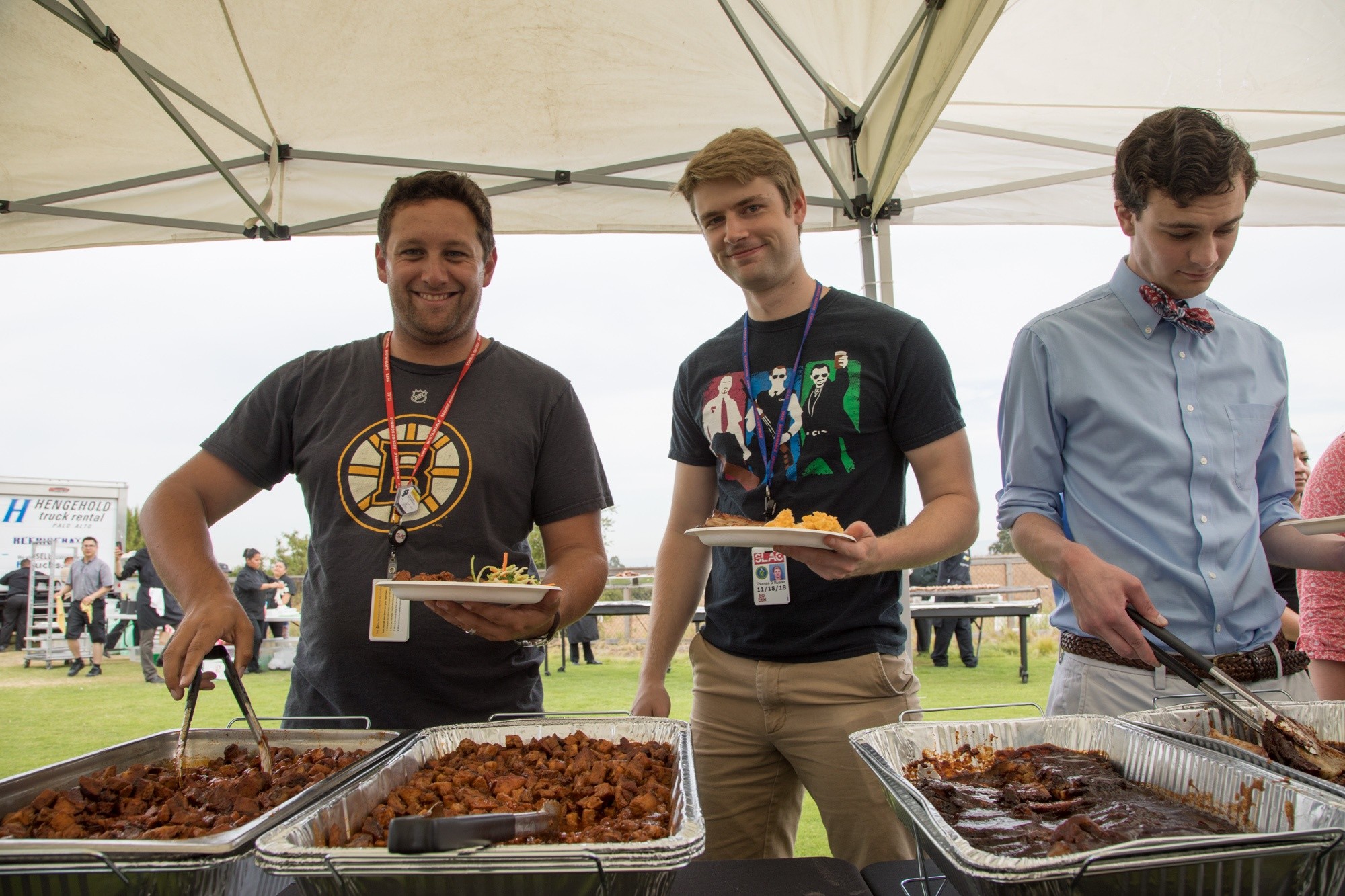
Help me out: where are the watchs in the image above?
[515,610,560,648]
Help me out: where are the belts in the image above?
[15,594,28,596]
[1060,628,1310,686]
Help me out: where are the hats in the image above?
[218,563,233,573]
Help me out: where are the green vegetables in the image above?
[495,571,539,585]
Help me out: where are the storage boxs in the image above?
[202,637,298,680]
[39,634,84,648]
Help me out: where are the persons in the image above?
[908,548,978,669]
[135,171,613,731]
[772,566,785,581]
[996,105,1344,714]
[630,127,980,868]
[0,537,296,684]
[566,616,602,666]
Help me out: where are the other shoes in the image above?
[935,659,947,666]
[68,662,84,676]
[147,674,165,683]
[86,666,102,676]
[104,651,111,659]
[587,660,602,664]
[967,662,977,668]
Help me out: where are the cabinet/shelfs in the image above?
[22,539,93,661]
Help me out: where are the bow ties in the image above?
[1137,282,1215,340]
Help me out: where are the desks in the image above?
[264,616,300,627]
[545,599,1041,684]
[104,613,138,651]
[276,856,958,896]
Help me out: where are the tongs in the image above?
[388,797,563,856]
[172,644,274,788]
[1123,605,1324,756]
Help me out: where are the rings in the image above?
[465,629,475,635]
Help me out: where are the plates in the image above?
[376,579,564,605]
[1278,514,1345,535]
[682,527,860,550]
